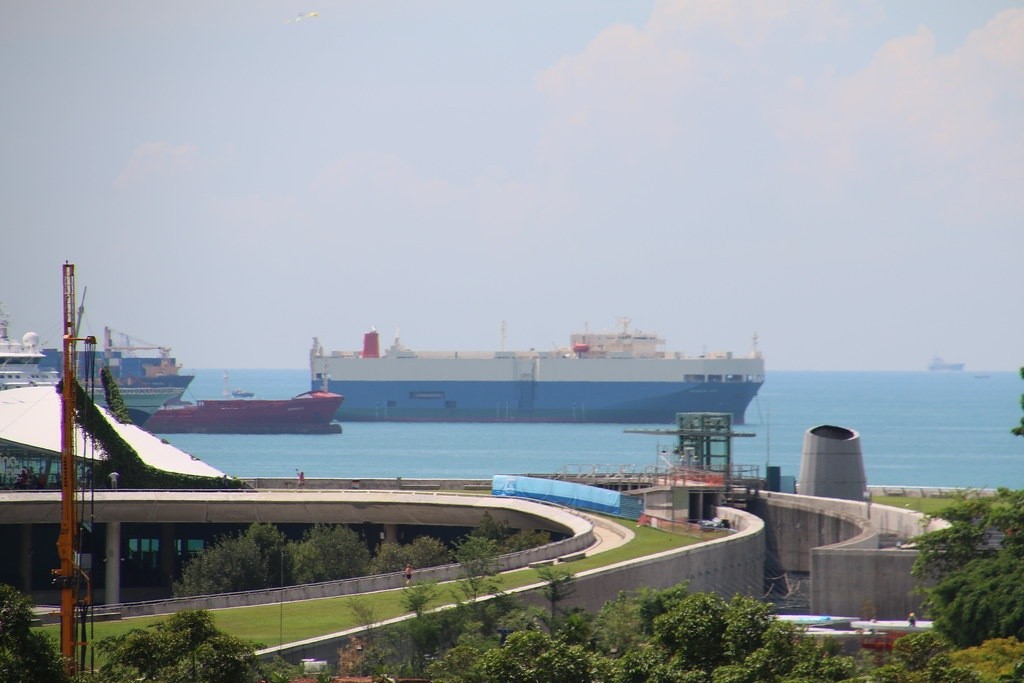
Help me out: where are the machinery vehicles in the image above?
[54,259,96,674]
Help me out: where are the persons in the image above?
[295,470,306,490]
[870,616,877,632]
[14,465,46,490]
[108,472,119,490]
[909,613,917,627]
[693,457,700,465]
[681,455,686,464]
[405,564,413,585]
[673,447,680,454]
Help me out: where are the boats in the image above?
[309,314,765,424]
[231,389,253,397]
[0,313,64,386]
[40,324,194,411]
[139,391,347,435]
[928,356,965,371]
